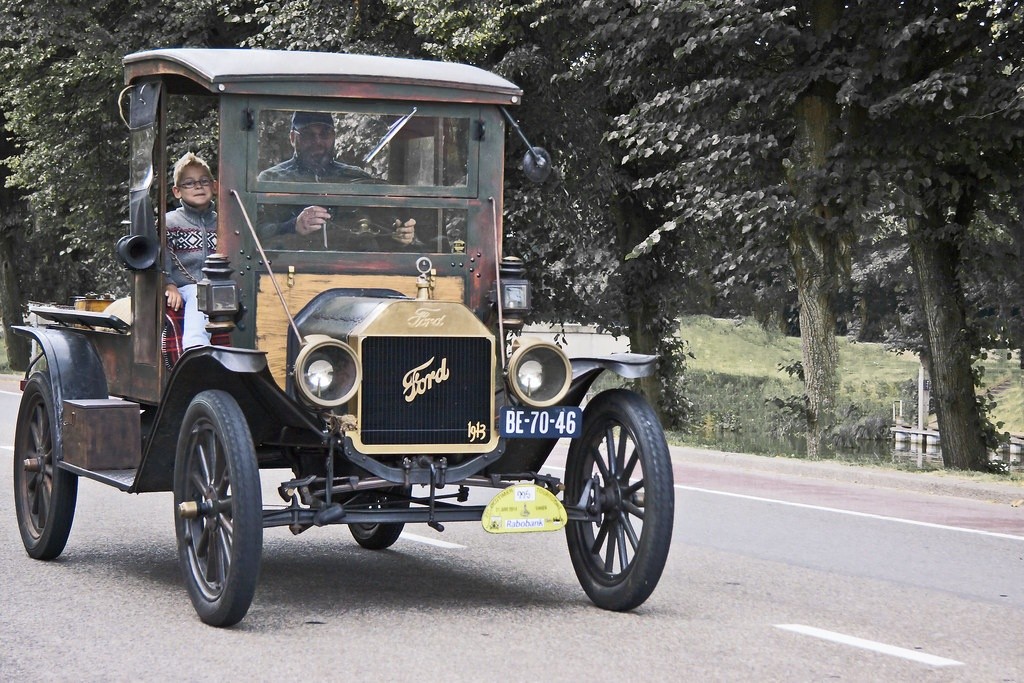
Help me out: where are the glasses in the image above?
[292,128,335,140]
[179,178,211,189]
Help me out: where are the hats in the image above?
[291,111,334,129]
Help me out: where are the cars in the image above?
[8,48,676,629]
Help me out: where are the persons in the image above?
[156,150,220,352]
[252,110,429,253]
